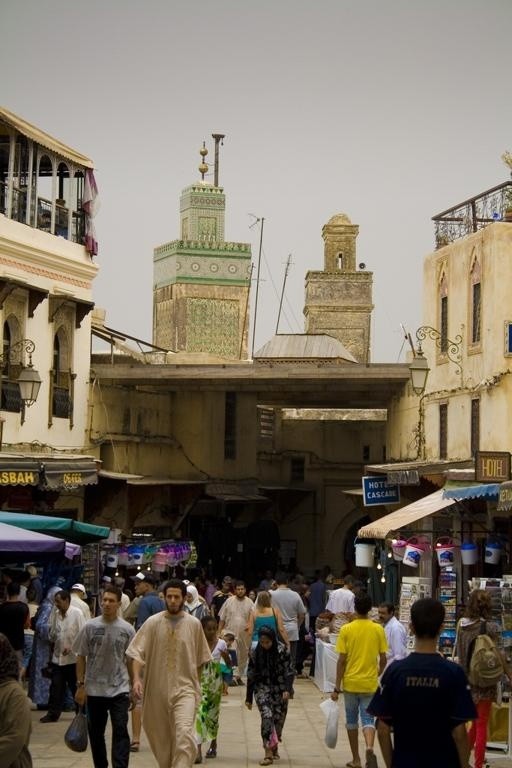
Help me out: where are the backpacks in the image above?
[465,620,504,687]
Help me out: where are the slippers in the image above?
[347,761,361,768]
[206,748,216,757]
[366,754,377,768]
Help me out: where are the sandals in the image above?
[273,752,280,759]
[130,742,140,751]
[260,757,273,765]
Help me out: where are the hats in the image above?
[129,571,156,582]
[102,576,112,582]
[223,576,232,584]
[72,583,88,600]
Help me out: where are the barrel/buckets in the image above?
[353,535,378,568]
[485,536,502,565]
[434,535,457,567]
[459,535,478,564]
[390,534,407,562]
[104,542,147,569]
[402,535,428,568]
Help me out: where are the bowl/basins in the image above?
[328,634,340,645]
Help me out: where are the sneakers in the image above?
[236,677,244,685]
[231,680,236,686]
[297,672,309,679]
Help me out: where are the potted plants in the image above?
[504,190,512,221]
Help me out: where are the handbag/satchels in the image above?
[41,668,53,678]
[279,633,286,644]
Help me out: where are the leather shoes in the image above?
[40,714,59,722]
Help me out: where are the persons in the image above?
[1,565,512,768]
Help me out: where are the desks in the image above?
[314,637,338,693]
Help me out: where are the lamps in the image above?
[0,339,43,408]
[409,326,462,396]
